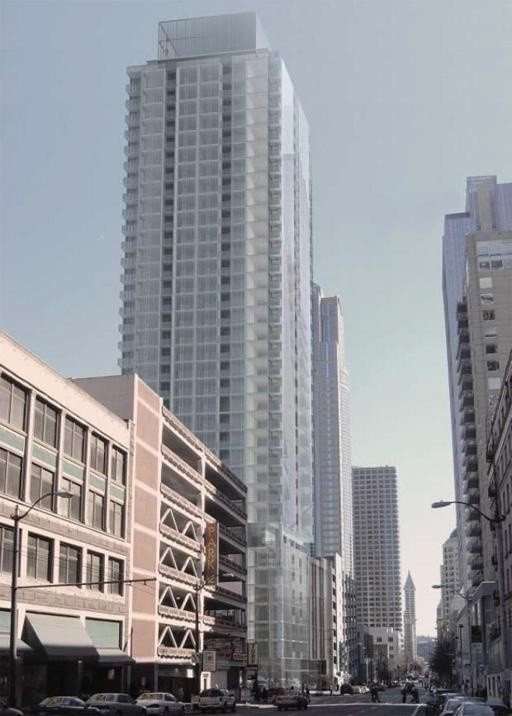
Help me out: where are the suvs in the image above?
[198,688,236,713]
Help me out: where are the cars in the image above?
[428,688,511,716]
[275,690,310,710]
[0,692,185,716]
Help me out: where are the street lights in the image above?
[195,573,233,696]
[11,491,73,708]
[433,501,508,688]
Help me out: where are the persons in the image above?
[400,679,430,704]
[305,687,310,704]
[251,682,269,704]
[474,683,488,704]
[369,679,381,704]
